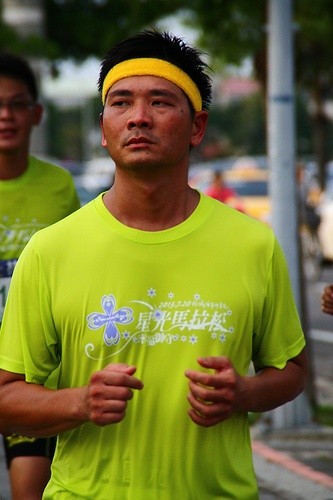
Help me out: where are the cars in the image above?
[205,154,274,223]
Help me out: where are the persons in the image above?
[0,50,80,500]
[321,284,333,318]
[202,167,243,212]
[315,194,333,281]
[1,27,307,500]
[303,176,324,263]
